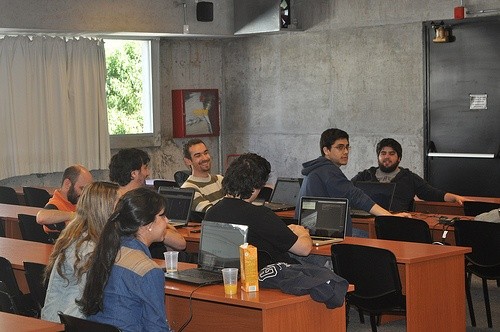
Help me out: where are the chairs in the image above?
[0,170,500,332]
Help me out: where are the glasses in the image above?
[330,145,352,150]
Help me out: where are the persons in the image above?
[41,182,122,323]
[294,128,412,236]
[351,138,471,213]
[203,152,313,265]
[109,148,186,250]
[36,165,93,245]
[180,139,228,214]
[75,188,173,332]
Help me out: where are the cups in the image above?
[164,251,178,272]
[221,268,239,295]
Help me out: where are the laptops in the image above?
[164,221,248,286]
[350,178,396,218]
[158,186,195,227]
[264,176,303,211]
[298,196,349,247]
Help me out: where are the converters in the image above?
[439,217,457,226]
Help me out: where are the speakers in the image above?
[196,2,214,21]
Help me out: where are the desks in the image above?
[1,203,472,331]
[414,194,499,215]
[0,237,355,332]
[272,209,475,247]
